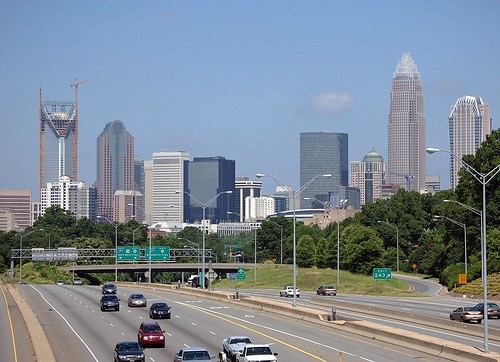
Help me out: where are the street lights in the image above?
[303,197,339,284]
[19,229,43,285]
[425,146,488,354]
[96,190,233,290]
[255,172,332,307]
[378,221,399,272]
[265,219,283,264]
[441,197,484,288]
[434,215,467,275]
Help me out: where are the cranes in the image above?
[389,171,416,192]
[69,78,89,181]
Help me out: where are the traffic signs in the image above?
[117,246,141,261]
[145,246,171,260]
[373,268,391,280]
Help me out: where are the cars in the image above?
[55,279,65,285]
[236,344,278,362]
[99,284,173,362]
[280,286,338,298]
[222,336,255,360]
[449,307,483,324]
[72,277,83,285]
[174,347,216,362]
[473,302,500,319]
